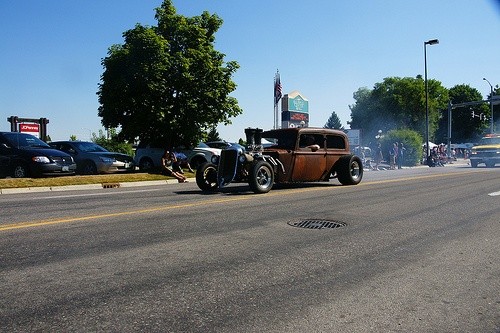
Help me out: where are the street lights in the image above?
[423,38,439,165]
[482,77,493,133]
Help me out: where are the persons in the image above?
[374,142,383,171]
[398,143,407,169]
[161,150,186,183]
[389,142,398,170]
[422,143,472,167]
[172,147,194,174]
[360,147,375,170]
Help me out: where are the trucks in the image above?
[349,147,375,166]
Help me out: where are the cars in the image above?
[135,137,223,174]
[0,131,77,179]
[46,141,135,176]
[204,141,275,154]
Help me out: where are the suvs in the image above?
[470,133,500,167]
[196,127,363,194]
